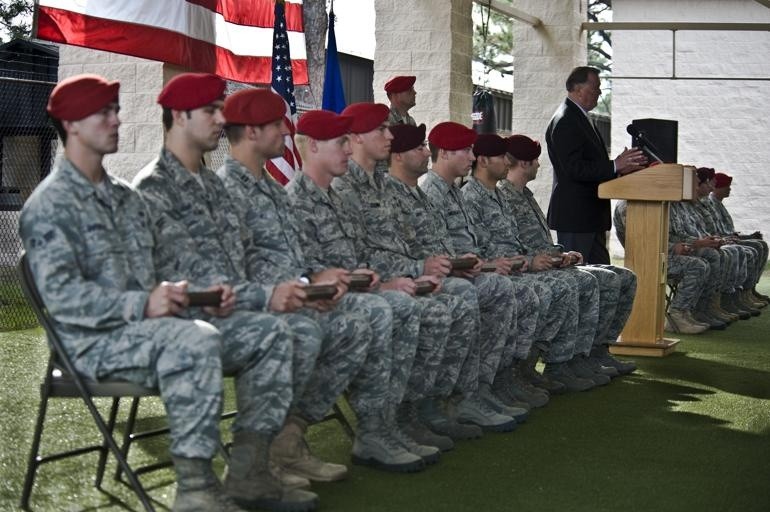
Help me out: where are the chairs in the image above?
[11,248,358,512]
[665,273,685,335]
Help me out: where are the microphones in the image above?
[626,123,665,161]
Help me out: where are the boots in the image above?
[519,343,638,393]
[350,364,549,471]
[663,288,769,334]
[171,417,347,512]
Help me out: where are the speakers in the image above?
[631,118,678,163]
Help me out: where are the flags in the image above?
[322,11,346,114]
[31,0,310,85]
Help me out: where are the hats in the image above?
[223,88,286,126]
[390,125,426,152]
[697,167,731,187]
[157,72,226,110]
[46,76,120,120]
[295,109,350,140]
[343,103,389,132]
[383,77,417,94]
[506,135,540,160]
[429,123,478,151]
[474,133,510,155]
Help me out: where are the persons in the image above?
[131,73,442,490]
[17,75,318,511]
[545,66,646,265]
[286,104,638,451]
[615,158,770,334]
